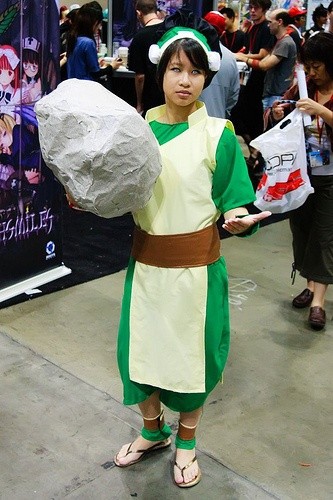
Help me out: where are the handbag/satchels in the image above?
[249,105,315,214]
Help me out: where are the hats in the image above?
[288,6,307,17]
[148,27,221,73]
[203,10,227,35]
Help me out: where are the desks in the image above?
[113,65,144,114]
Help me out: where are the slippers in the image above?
[173,454,201,487]
[113,437,171,466]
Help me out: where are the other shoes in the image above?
[292,288,315,308]
[308,306,326,328]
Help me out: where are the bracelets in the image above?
[252,59,259,68]
[232,214,260,238]
[246,57,250,65]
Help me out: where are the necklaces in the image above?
[144,18,158,26]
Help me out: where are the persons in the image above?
[58,0,333,171]
[265,32,333,328]
[65,26,272,488]
